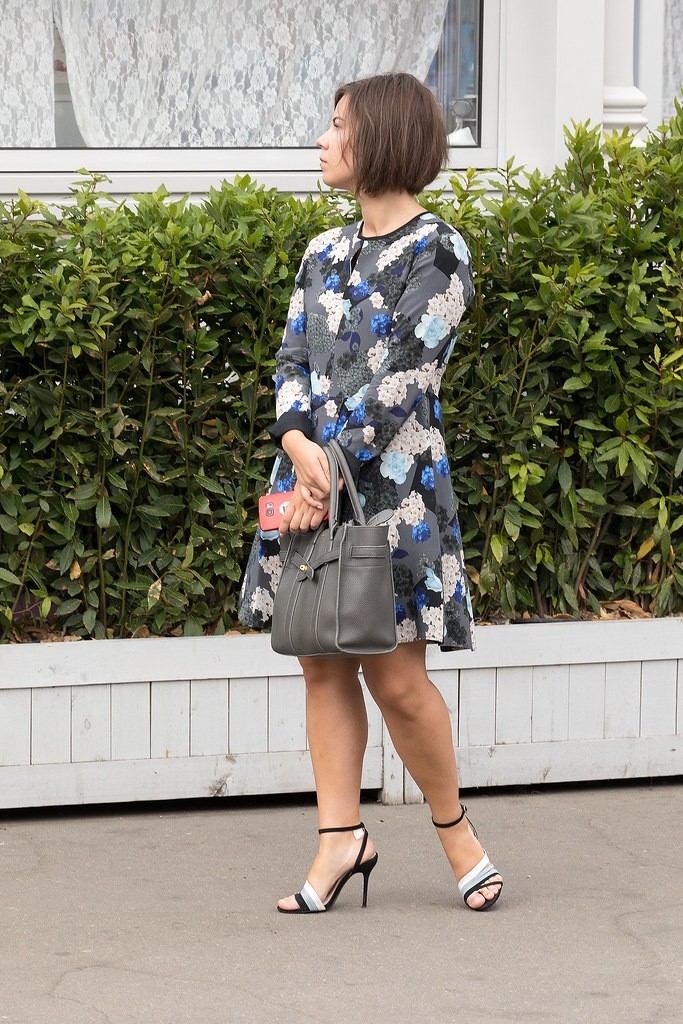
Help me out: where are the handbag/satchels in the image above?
[271,439,398,659]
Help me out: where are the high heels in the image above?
[432,804,503,911]
[277,822,378,913]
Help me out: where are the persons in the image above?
[239,74,502,913]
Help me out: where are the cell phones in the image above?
[258,490,330,531]
